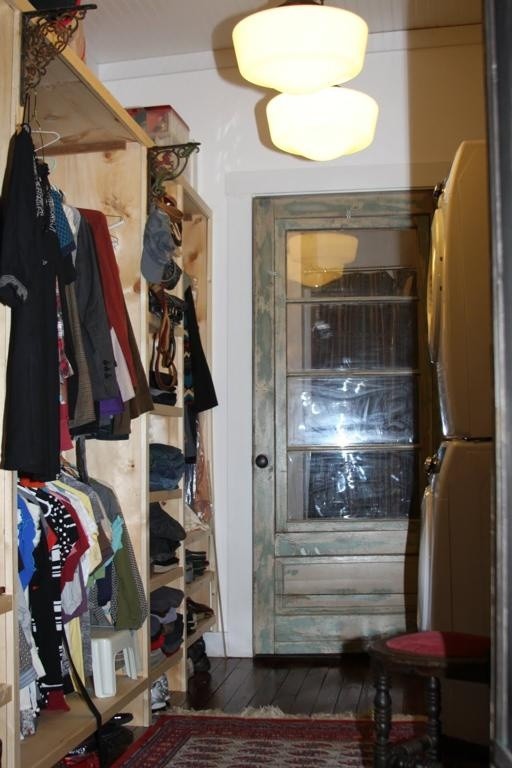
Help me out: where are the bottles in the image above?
[140,210,179,286]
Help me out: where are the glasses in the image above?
[264,90,379,162]
[232,0,378,98]
[285,231,358,288]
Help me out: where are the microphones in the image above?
[88,625,137,698]
[371,631,491,768]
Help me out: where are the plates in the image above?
[0,3,219,768]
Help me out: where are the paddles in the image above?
[110,706,431,768]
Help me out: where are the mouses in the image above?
[30,130,60,152]
[101,214,123,227]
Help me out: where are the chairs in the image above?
[151,683,166,711]
[156,673,171,702]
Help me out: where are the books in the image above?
[124,105,190,177]
